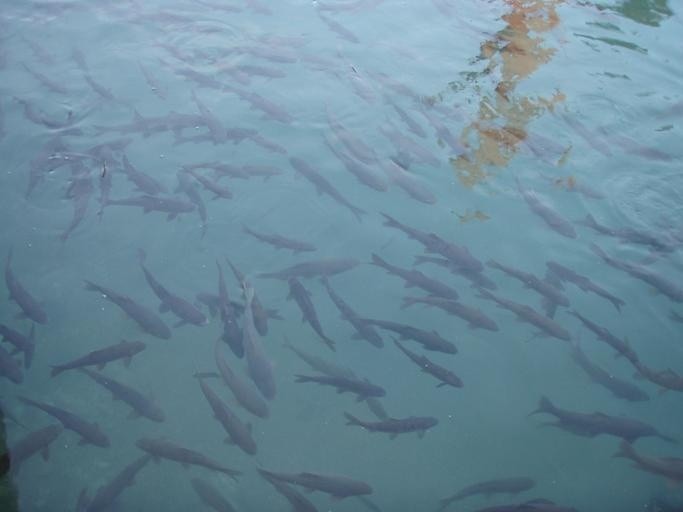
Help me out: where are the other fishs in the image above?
[0,0,683,512]
